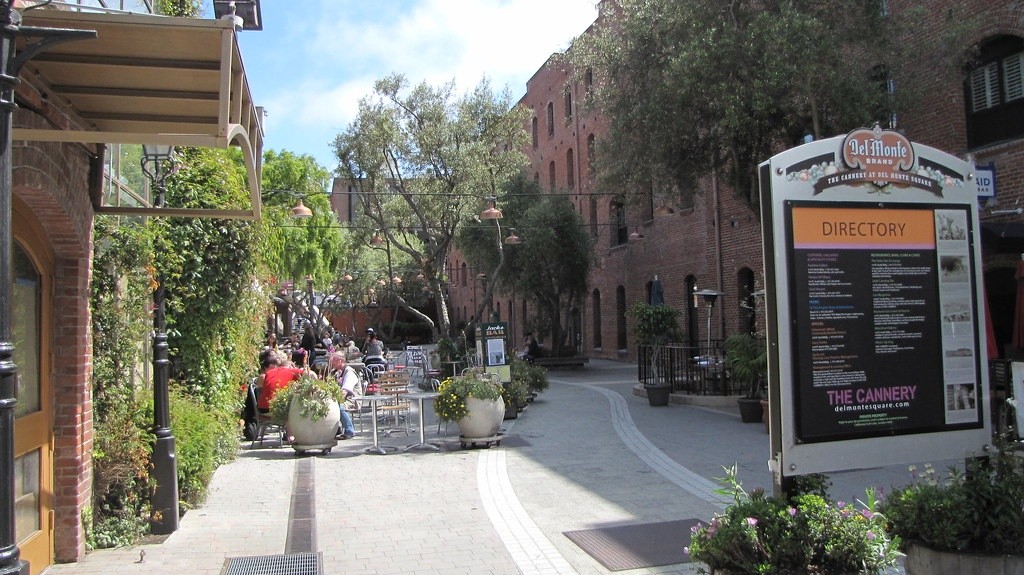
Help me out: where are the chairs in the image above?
[248,333,472,456]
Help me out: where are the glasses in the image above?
[366,334,371,335]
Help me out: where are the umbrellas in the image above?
[983,258,1024,361]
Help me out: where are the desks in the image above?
[329,363,373,394]
[313,347,327,356]
[353,395,399,455]
[442,361,460,380]
[375,371,395,377]
[401,392,440,452]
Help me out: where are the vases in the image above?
[456,393,505,447]
[286,393,341,455]
[504,405,517,419]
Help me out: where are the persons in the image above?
[517,333,538,361]
[257,322,389,440]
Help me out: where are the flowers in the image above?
[501,356,551,407]
[265,376,344,423]
[433,368,506,423]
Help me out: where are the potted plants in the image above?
[625,301,683,405]
[722,334,767,423]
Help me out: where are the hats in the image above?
[365,328,374,333]
[346,340,355,345]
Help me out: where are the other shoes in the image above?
[334,433,355,439]
[282,433,287,440]
